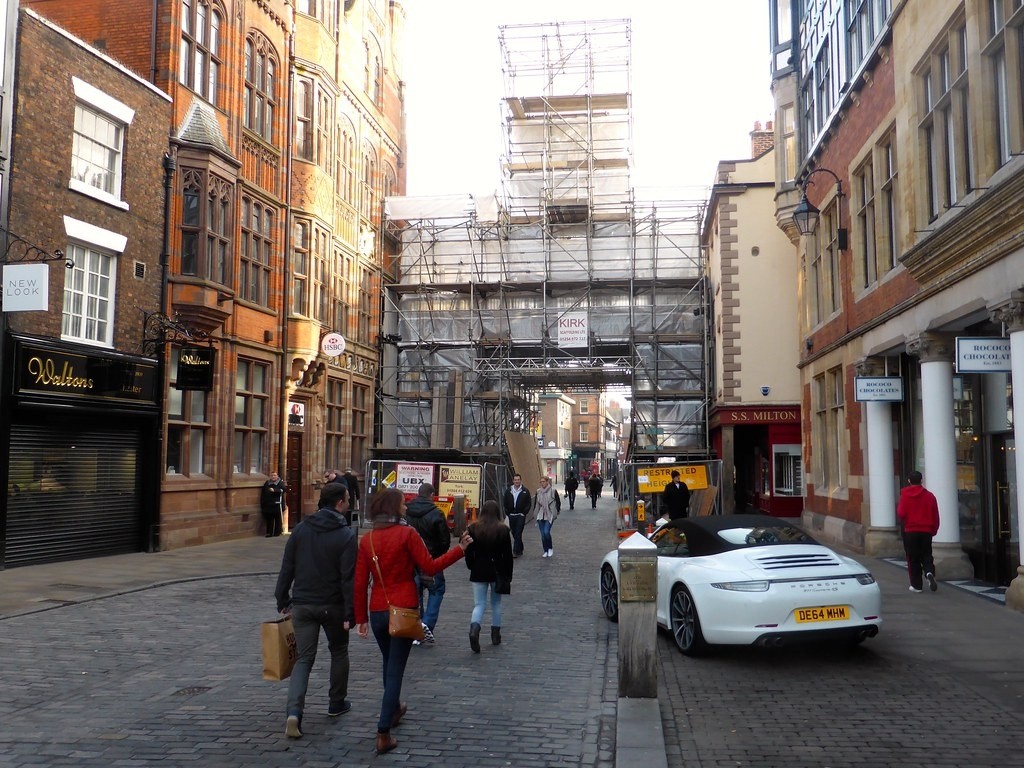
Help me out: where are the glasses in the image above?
[325,474,330,478]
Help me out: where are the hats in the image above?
[671,470,680,479]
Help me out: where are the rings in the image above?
[465,539,469,542]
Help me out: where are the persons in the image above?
[572,466,578,482]
[343,467,360,527]
[584,471,592,497]
[581,468,586,481]
[597,474,603,497]
[504,473,531,558]
[465,500,514,653]
[533,476,561,558]
[896,471,940,592]
[324,468,348,488]
[564,471,578,509]
[260,471,287,537]
[273,483,358,737]
[662,470,690,520]
[353,488,473,753]
[610,476,617,499]
[404,483,450,643]
[588,473,601,508]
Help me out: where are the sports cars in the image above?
[597,514,882,659]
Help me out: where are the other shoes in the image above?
[285,711,304,738]
[328,700,351,716]
[513,552,524,557]
[265,534,273,537]
[542,552,547,557]
[909,586,923,593]
[413,623,436,645]
[274,534,279,536]
[926,572,938,591]
[548,548,553,557]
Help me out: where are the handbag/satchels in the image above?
[495,573,511,595]
[261,612,297,682]
[388,606,425,641]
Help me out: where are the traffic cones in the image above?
[623,507,632,529]
[645,522,654,539]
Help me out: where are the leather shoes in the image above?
[393,701,408,726]
[376,732,397,753]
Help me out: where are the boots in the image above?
[491,626,502,644]
[468,623,481,653]
